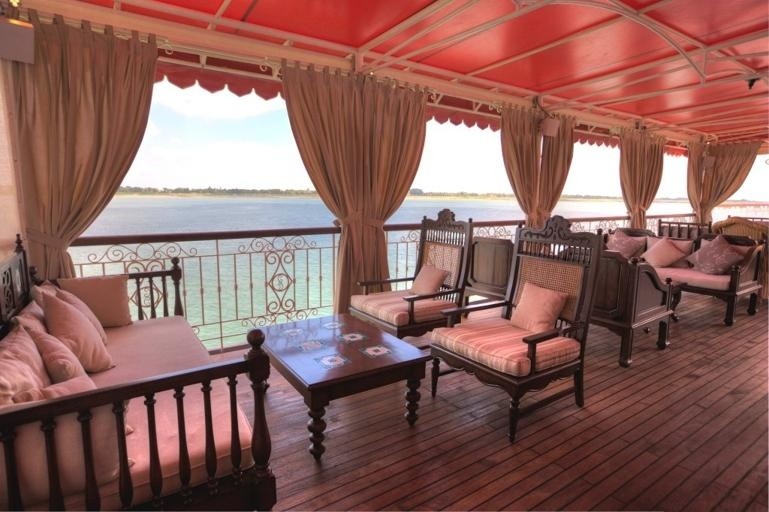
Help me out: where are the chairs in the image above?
[710,215,769,301]
[348,207,600,445]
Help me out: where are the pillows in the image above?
[1,271,135,509]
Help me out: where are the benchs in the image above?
[2,233,278,510]
[462,220,766,371]
